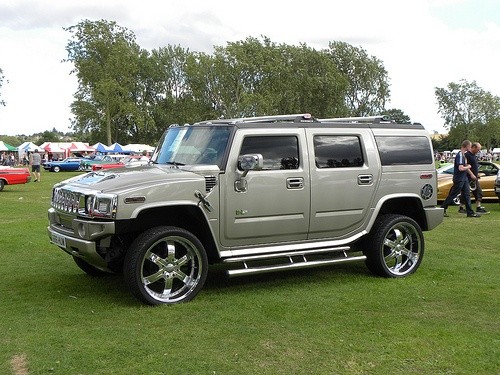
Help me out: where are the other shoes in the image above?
[444,209,449,217]
[34,179,37,182]
[467,213,481,217]
[458,209,466,215]
[476,206,490,215]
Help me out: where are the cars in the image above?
[42,157,92,173]
[78,154,153,173]
[436,160,500,206]
[0,165,32,192]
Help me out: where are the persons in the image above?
[43,152,54,161]
[440,140,481,217]
[23,152,33,165]
[458,141,490,215]
[55,153,63,161]
[32,149,41,183]
[72,152,106,160]
[114,152,151,161]
[0,151,17,165]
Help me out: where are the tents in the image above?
[0,141,154,157]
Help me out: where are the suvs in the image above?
[46,112,444,307]
